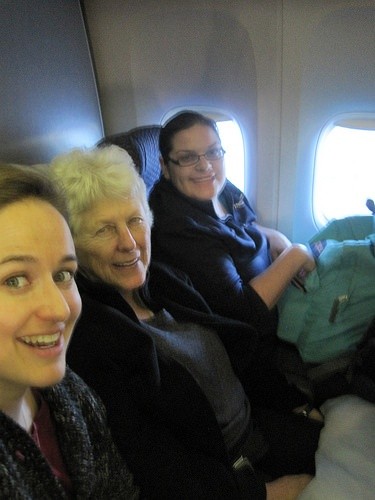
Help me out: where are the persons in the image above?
[46,144,325,500]
[148,109,375,405]
[0,163,140,500]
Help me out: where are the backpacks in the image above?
[275,200,374,364]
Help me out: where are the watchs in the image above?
[300,405,314,417]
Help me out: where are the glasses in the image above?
[167,146,226,167]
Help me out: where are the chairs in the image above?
[97,123,356,395]
